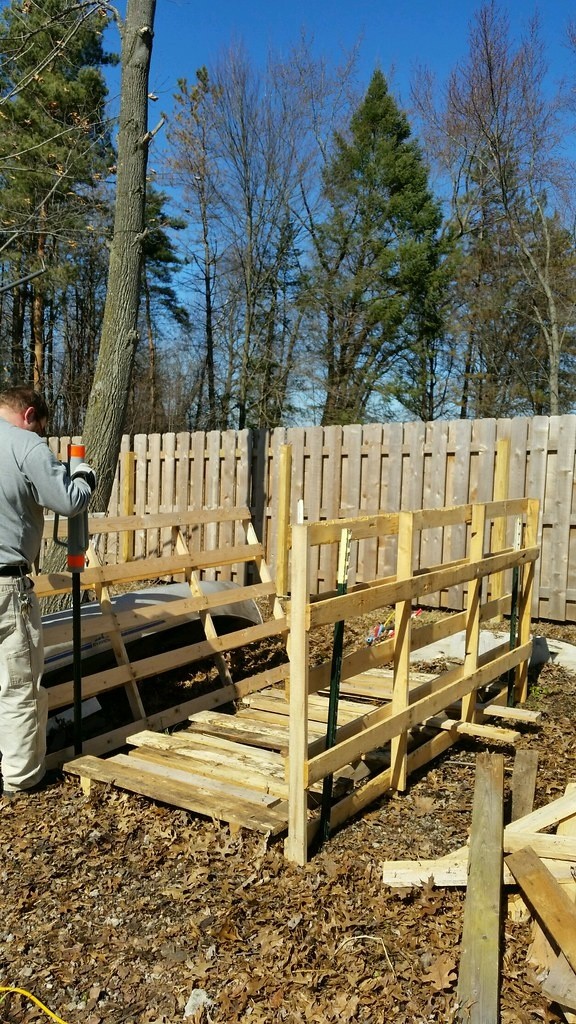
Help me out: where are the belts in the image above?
[0,565,28,576]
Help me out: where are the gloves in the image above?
[69,462,98,491]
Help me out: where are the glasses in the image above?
[35,415,45,437]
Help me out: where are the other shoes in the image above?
[4,767,62,797]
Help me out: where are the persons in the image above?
[0,387,97,798]
